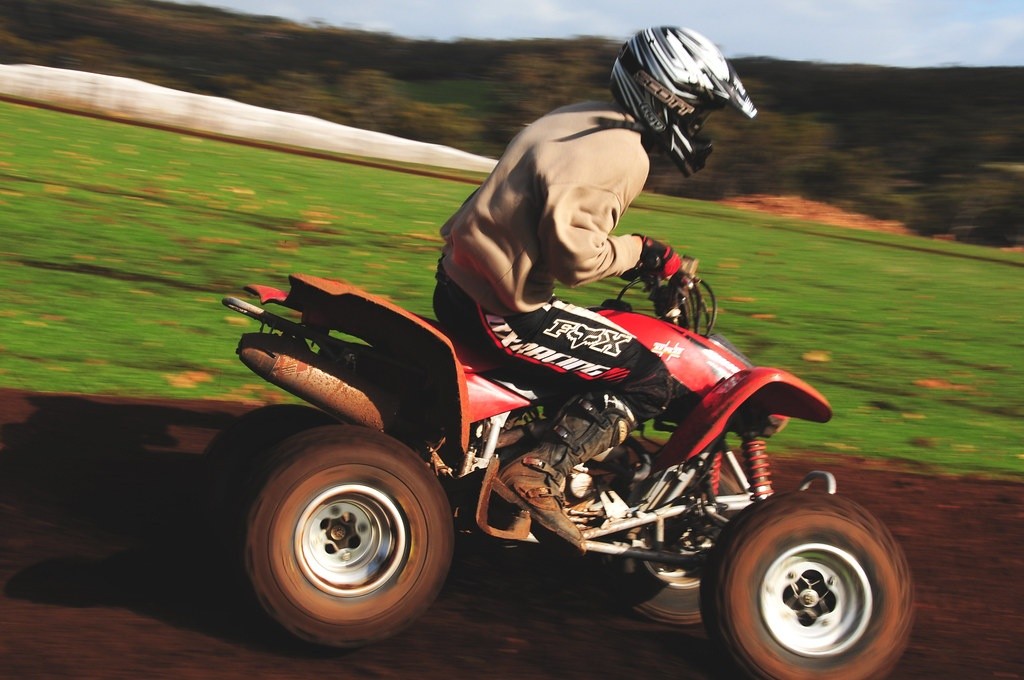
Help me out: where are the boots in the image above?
[489,389,637,557]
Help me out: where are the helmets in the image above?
[610,25,758,180]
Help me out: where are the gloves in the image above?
[620,233,703,307]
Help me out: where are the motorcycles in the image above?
[221,253,916,680]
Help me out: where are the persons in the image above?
[433,27,758,546]
